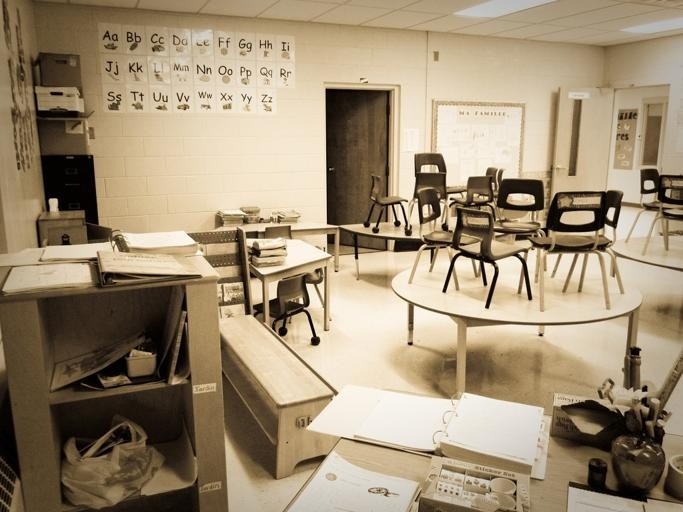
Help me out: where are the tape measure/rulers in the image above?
[656,351,683,420]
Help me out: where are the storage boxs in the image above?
[36,109,96,155]
[32,51,83,98]
[35,85,84,112]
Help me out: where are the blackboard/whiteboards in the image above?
[431,99,525,189]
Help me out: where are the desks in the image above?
[340,218,547,280]
[293,432,682,512]
[246,238,333,331]
[211,224,341,272]
[390,247,643,399]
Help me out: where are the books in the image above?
[217,210,246,227]
[353,392,544,475]
[272,210,301,224]
[110,230,198,257]
[156,285,190,385]
[49,328,146,392]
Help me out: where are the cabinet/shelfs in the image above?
[38,210,112,245]
[41,155,99,224]
[189,227,254,314]
[1,243,229,512]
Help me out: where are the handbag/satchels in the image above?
[63,414,165,508]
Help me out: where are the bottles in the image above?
[588,458,607,488]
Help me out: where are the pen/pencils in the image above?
[631,385,673,446]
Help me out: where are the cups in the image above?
[490,493,516,510]
[490,477,517,495]
[48,198,59,212]
[666,455,683,501]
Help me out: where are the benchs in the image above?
[220,314,339,480]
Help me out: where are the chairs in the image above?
[265,225,292,238]
[253,273,320,346]
[442,207,534,309]
[283,245,332,324]
[625,168,682,257]
[405,155,624,296]
[365,174,412,234]
[527,191,611,310]
